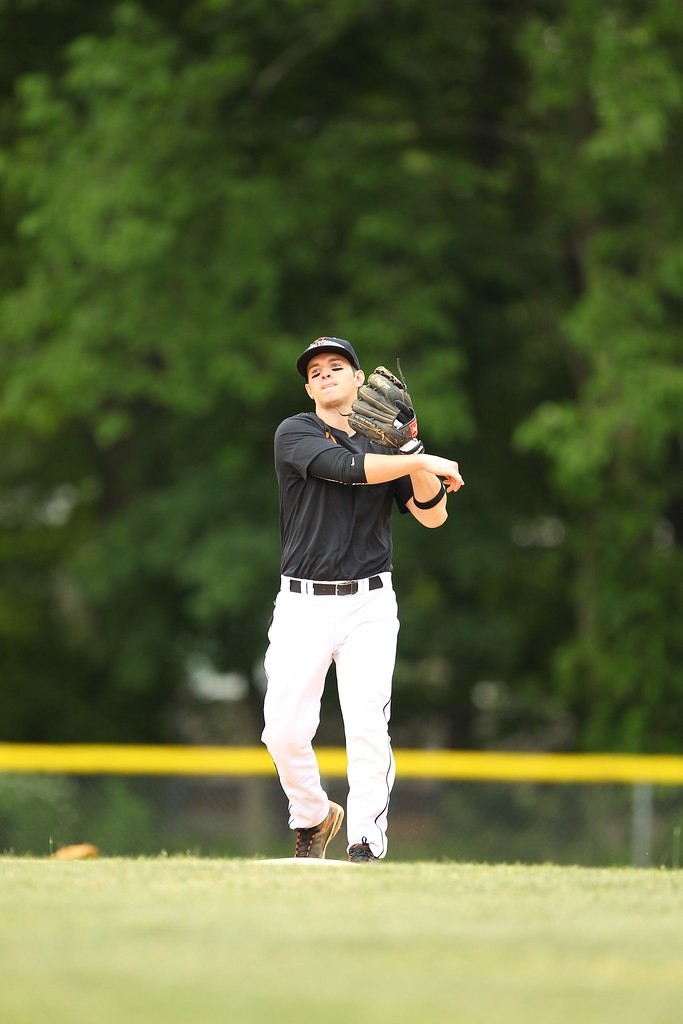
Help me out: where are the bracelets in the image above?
[412,478,446,510]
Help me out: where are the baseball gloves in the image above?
[347,357,420,451]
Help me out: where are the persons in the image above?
[261,335,465,867]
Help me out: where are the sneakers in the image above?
[292,800,343,859]
[348,836,380,865]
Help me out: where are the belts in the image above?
[289,575,384,596]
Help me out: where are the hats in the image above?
[294,336,361,384]
